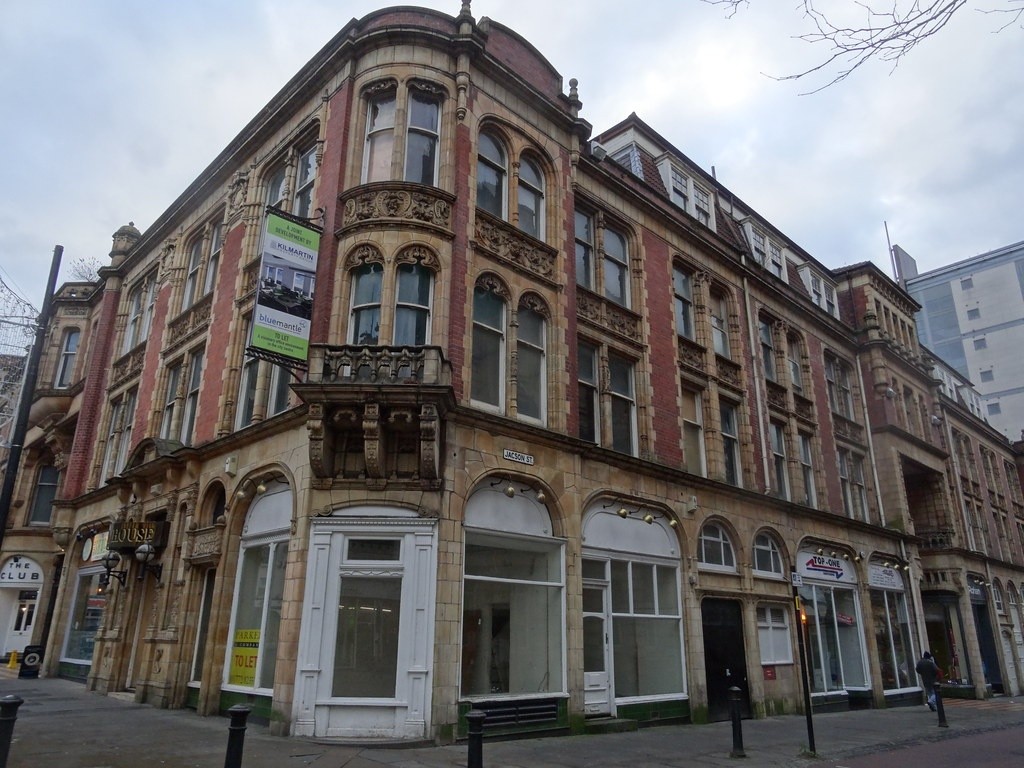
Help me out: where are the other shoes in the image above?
[926,701,937,712]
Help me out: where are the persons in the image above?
[916,652,939,712]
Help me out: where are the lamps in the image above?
[520,480,545,504]
[877,556,909,571]
[931,414,940,425]
[491,471,514,498]
[629,504,652,525]
[802,539,824,555]
[653,507,677,529]
[849,550,860,563]
[970,576,990,588]
[886,387,896,398]
[256,472,290,495]
[134,539,163,582]
[236,478,258,500]
[101,550,128,587]
[821,542,836,558]
[603,495,626,519]
[837,546,849,561]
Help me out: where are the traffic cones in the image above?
[7,649,19,668]
